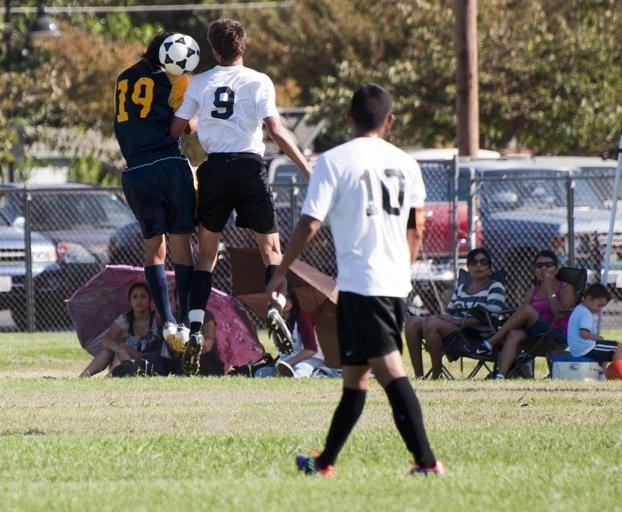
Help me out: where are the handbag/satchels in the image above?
[112,358,151,376]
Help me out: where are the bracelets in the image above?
[548,292,557,300]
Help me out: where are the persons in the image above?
[172,287,225,376]
[405,247,505,378]
[170,19,311,378]
[462,250,576,379]
[567,282,622,364]
[265,86,443,480]
[79,283,162,378]
[253,284,325,378]
[114,31,196,353]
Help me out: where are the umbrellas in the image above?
[65,266,265,378]
[229,246,340,371]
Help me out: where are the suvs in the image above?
[1,183,141,330]
[408,145,621,332]
[107,152,341,293]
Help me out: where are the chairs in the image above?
[420,268,504,381]
[493,267,588,381]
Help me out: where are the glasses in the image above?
[469,259,489,266]
[535,262,554,268]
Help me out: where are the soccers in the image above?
[158,34,200,75]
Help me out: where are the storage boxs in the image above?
[551,355,600,381]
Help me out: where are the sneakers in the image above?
[464,342,493,356]
[403,461,445,476]
[494,373,505,379]
[296,456,336,478]
[163,309,295,377]
